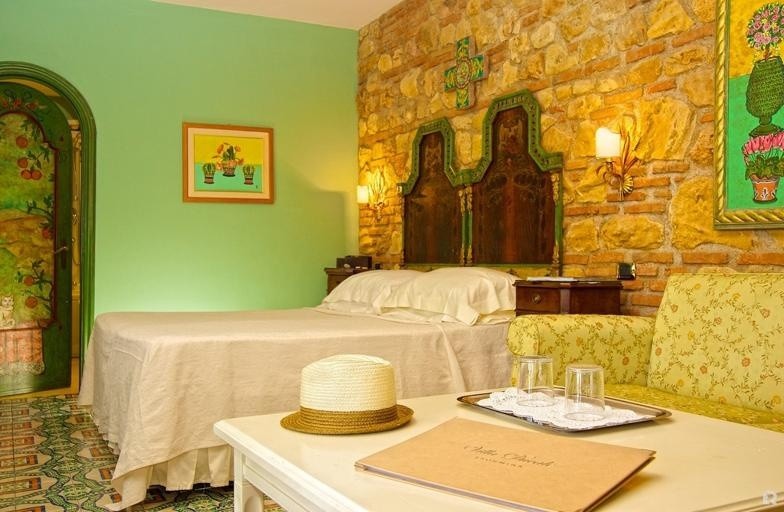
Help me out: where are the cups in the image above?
[513,353,559,408]
[562,362,611,424]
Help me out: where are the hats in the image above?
[278,350,416,439]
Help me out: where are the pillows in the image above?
[310,268,523,327]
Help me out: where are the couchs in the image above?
[506,272,783,434]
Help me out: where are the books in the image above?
[353,414,655,512]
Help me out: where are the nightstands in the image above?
[511,279,623,317]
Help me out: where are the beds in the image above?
[90,307,513,496]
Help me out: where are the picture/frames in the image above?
[712,0,784,231]
[182,122,275,205]
